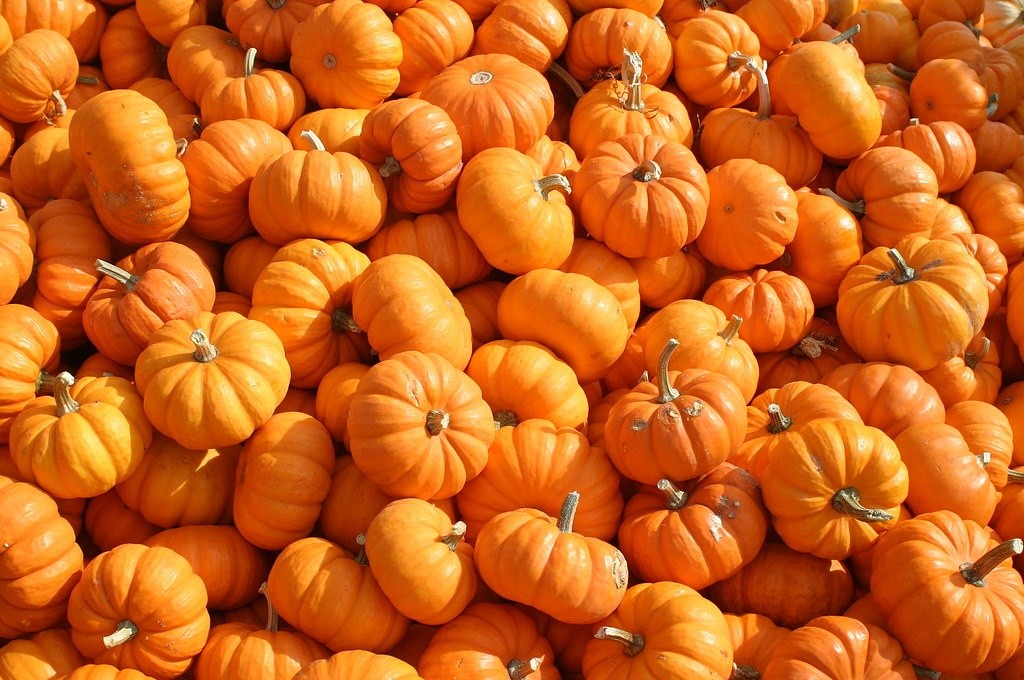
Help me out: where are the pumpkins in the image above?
[0,0,1024,680]
